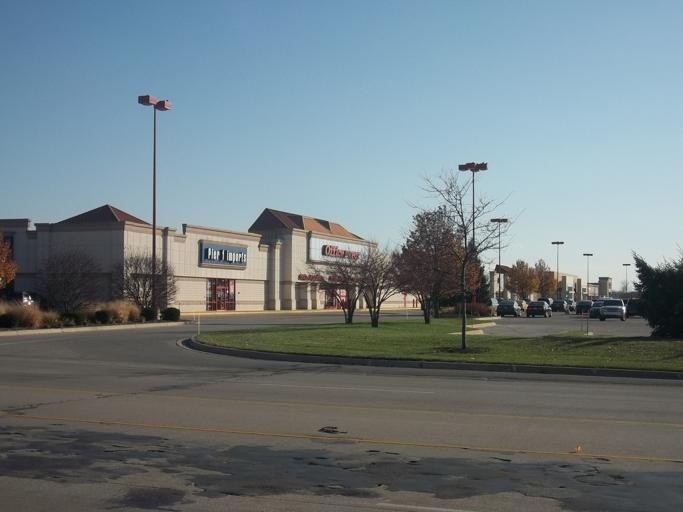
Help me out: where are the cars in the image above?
[0,291,42,310]
[455,296,647,321]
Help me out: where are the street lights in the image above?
[490,218,507,303]
[551,241,563,300]
[458,162,487,317]
[622,263,630,297]
[582,253,592,300]
[137,94,172,324]
